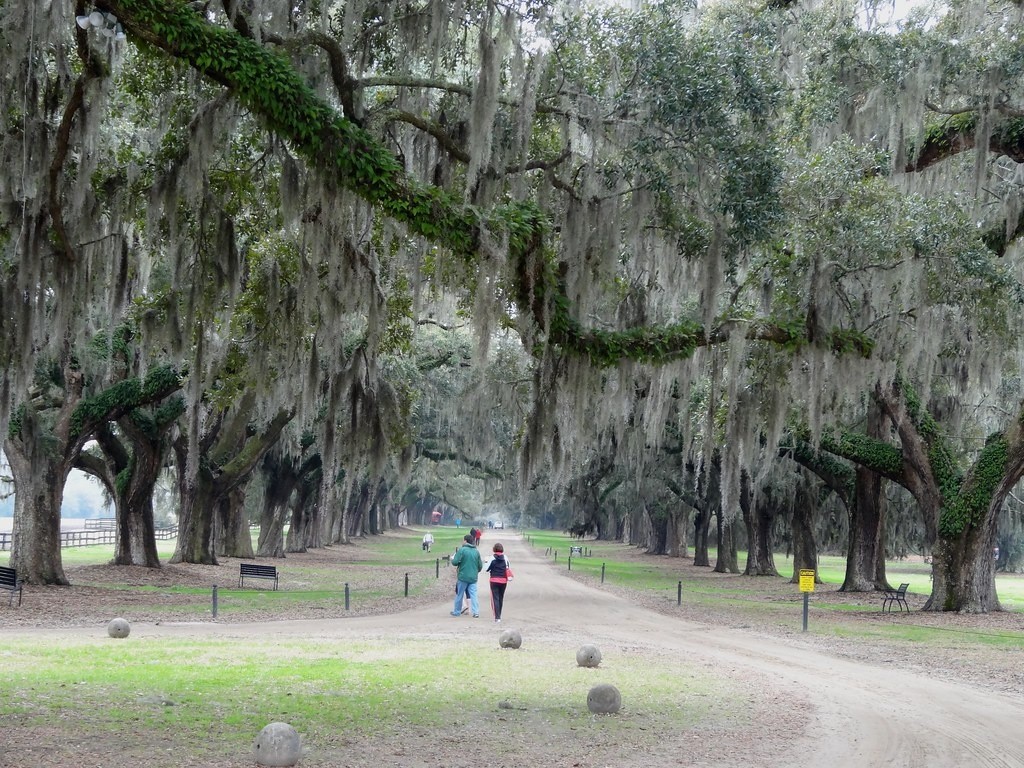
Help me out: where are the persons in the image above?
[423,531,434,554]
[484,542,513,622]
[470,527,481,545]
[489,520,494,528]
[455,517,460,528]
[451,534,482,617]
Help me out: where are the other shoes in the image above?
[472,614,479,618]
[450,612,460,616]
[428,551,430,552]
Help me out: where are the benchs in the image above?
[238,563,279,591]
[0,566,23,607]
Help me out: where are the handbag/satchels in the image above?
[506,568,514,581]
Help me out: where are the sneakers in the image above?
[492,619,501,626]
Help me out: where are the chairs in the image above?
[880,583,910,613]
